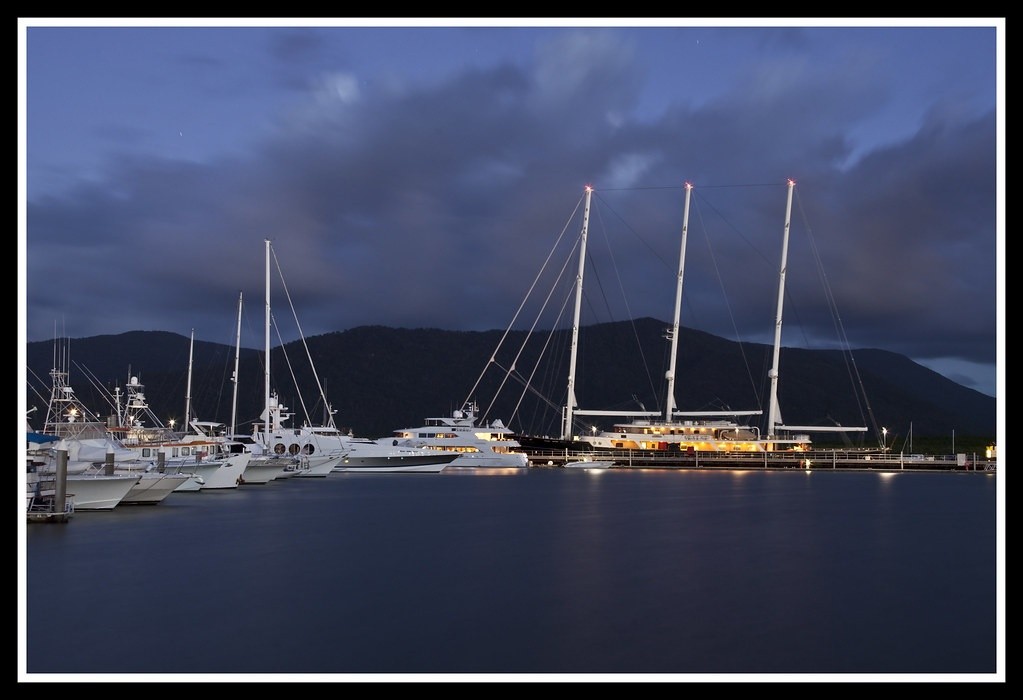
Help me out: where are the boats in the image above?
[27,406,76,522]
[564,460,615,470]
[26,315,154,511]
[331,431,462,471]
[108,361,227,492]
[379,411,531,469]
[71,354,194,503]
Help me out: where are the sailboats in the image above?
[176,233,353,489]
[460,179,891,466]
